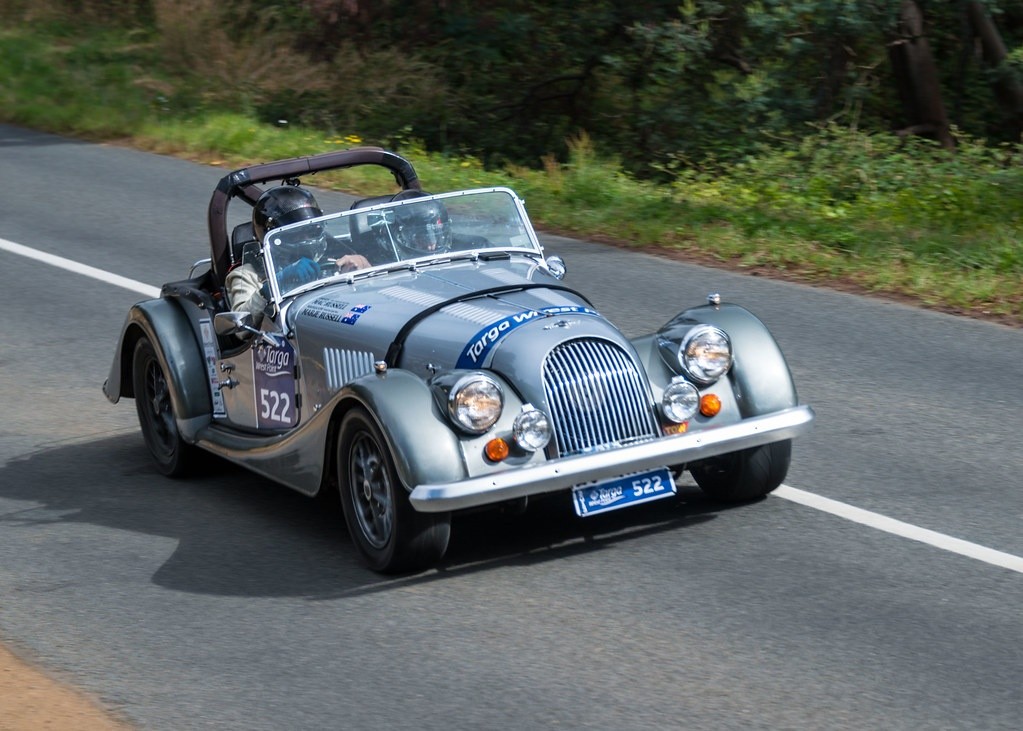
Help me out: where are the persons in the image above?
[225,185,372,344]
[380,190,453,262]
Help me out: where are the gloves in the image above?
[260,256,323,296]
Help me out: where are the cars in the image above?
[102,146,818,581]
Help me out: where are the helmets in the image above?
[372,189,453,261]
[253,186,328,263]
